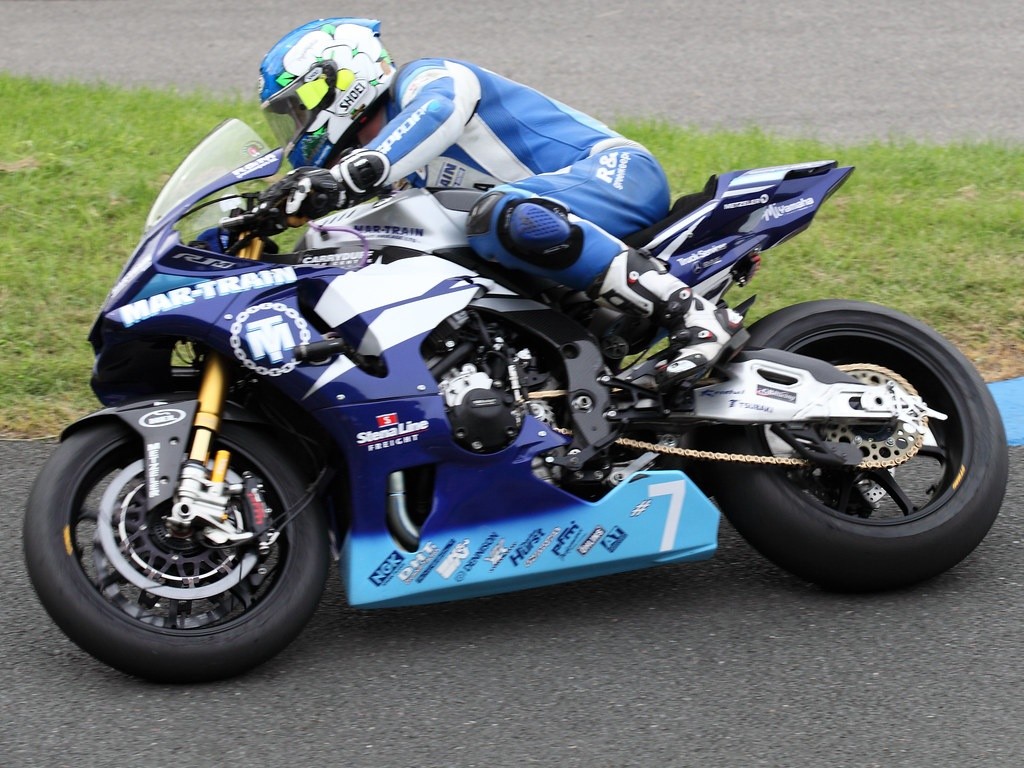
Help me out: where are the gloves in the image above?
[254,166,347,233]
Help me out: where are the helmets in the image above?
[258,15,397,175]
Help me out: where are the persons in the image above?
[196,15,741,388]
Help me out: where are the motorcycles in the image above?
[22,118,1010,690]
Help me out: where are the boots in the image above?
[589,247,750,385]
